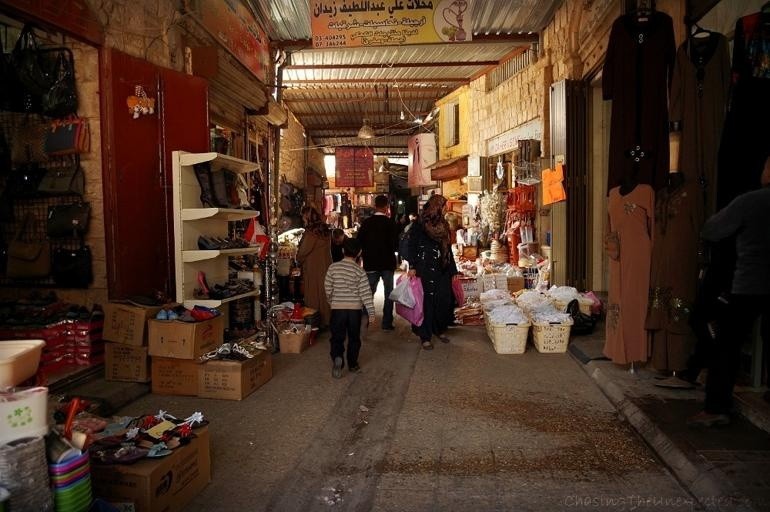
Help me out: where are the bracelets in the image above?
[697,251,704,256]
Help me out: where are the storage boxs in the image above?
[88,425,212,512]
[102,294,274,401]
[278,332,309,354]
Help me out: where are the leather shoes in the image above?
[198,234,252,251]
[192,270,257,300]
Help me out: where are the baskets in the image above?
[480,288,594,355]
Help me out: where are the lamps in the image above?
[356,118,376,139]
[400,103,405,120]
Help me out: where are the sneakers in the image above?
[382,325,395,334]
[331,356,343,379]
[0,290,106,333]
[421,340,433,350]
[349,364,360,372]
[154,303,223,323]
[437,333,450,344]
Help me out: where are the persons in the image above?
[643,172,706,381]
[395,211,405,228]
[323,238,375,378]
[681,158,770,430]
[605,164,657,374]
[407,193,460,350]
[357,194,399,331]
[329,227,362,266]
[295,205,334,332]
[401,212,420,241]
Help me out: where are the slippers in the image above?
[89,408,210,465]
[197,338,268,364]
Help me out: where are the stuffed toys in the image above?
[127,84,157,121]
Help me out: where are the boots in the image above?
[192,162,255,210]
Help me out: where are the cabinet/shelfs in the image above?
[172,149,263,344]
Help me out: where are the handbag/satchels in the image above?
[395,274,425,328]
[44,110,92,157]
[50,236,94,289]
[6,161,55,202]
[7,211,50,279]
[10,110,49,166]
[451,274,465,309]
[37,152,86,197]
[0,23,80,118]
[46,194,92,240]
